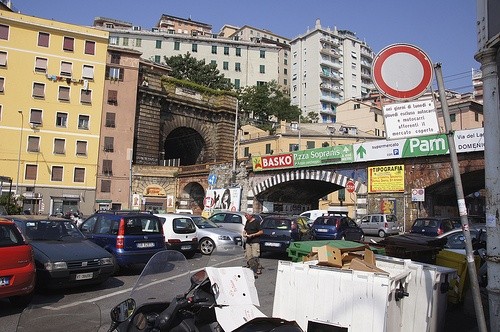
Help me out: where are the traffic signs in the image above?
[352,132,452,162]
[292,144,354,168]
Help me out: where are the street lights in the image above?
[14,110,24,194]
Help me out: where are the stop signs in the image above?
[345,181,356,193]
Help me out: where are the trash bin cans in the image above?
[382,233,449,265]
[286,240,386,262]
[437,249,468,306]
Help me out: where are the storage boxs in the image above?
[302,252,318,263]
[342,252,364,263]
[364,248,376,266]
[350,257,389,274]
[309,245,342,268]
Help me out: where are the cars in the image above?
[258,214,316,259]
[141,212,199,260]
[197,212,258,250]
[242,212,285,249]
[408,217,456,237]
[0,217,38,309]
[310,215,365,243]
[76,208,170,275]
[178,214,245,256]
[0,214,119,294]
[435,229,488,287]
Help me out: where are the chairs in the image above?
[176,219,182,226]
[224,214,233,222]
[428,220,436,227]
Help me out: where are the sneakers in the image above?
[256,270,260,273]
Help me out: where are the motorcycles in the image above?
[104,249,306,332]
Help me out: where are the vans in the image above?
[355,213,399,238]
[298,210,347,228]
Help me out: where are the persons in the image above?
[152,208,158,214]
[219,189,230,209]
[243,211,263,278]
